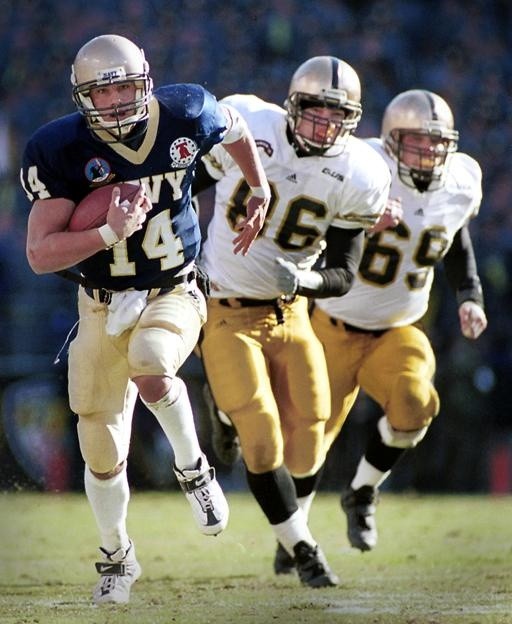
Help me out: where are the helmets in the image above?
[379,88,461,193]
[285,52,362,158]
[67,32,153,144]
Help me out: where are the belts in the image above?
[220,296,296,324]
[54,269,195,303]
[331,314,388,338]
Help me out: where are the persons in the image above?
[273,84,491,567]
[15,31,272,608]
[199,53,390,590]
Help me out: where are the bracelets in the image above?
[249,186,271,200]
[99,225,120,250]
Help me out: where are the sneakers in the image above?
[93,539,142,605]
[272,540,296,574]
[290,541,338,589]
[201,380,241,467]
[340,481,379,552]
[172,452,230,536]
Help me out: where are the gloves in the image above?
[276,258,299,295]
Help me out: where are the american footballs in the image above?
[68,184,140,231]
